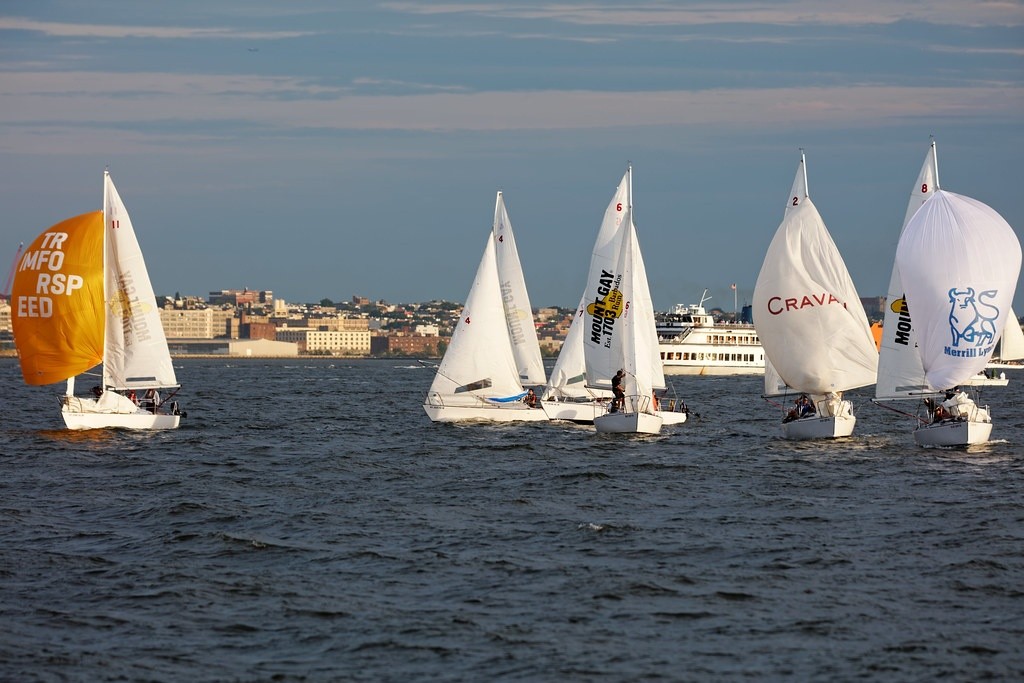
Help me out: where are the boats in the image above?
[659,315,766,377]
[944,370,1010,388]
[590,364,665,434]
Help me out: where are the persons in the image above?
[607,401,618,413]
[992,369,998,379]
[652,389,662,411]
[146,389,159,412]
[1000,371,1005,379]
[128,390,139,406]
[528,389,537,408]
[923,386,959,424]
[984,369,990,378]
[611,370,626,406]
[794,397,815,413]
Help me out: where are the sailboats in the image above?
[423,188,551,427]
[874,134,1023,448]
[982,306,1024,368]
[57,169,185,434]
[751,147,881,442]
[541,160,695,422]
